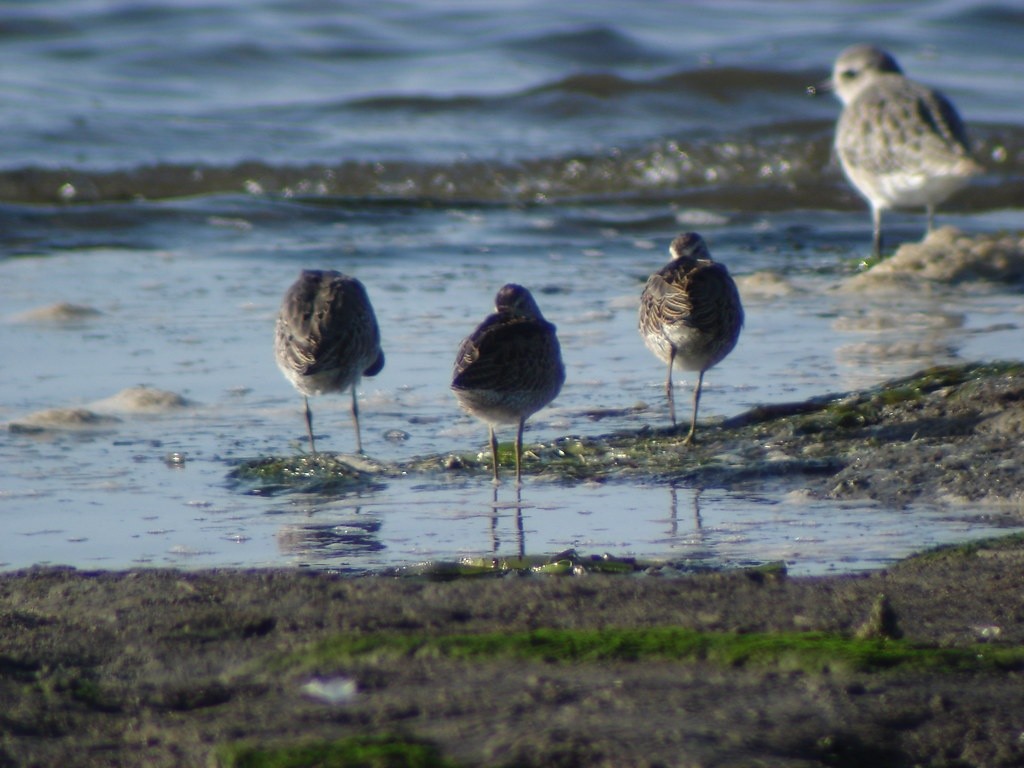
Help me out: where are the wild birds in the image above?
[807,44,988,261]
[448,282,566,489]
[639,233,745,447]
[271,268,385,459]
